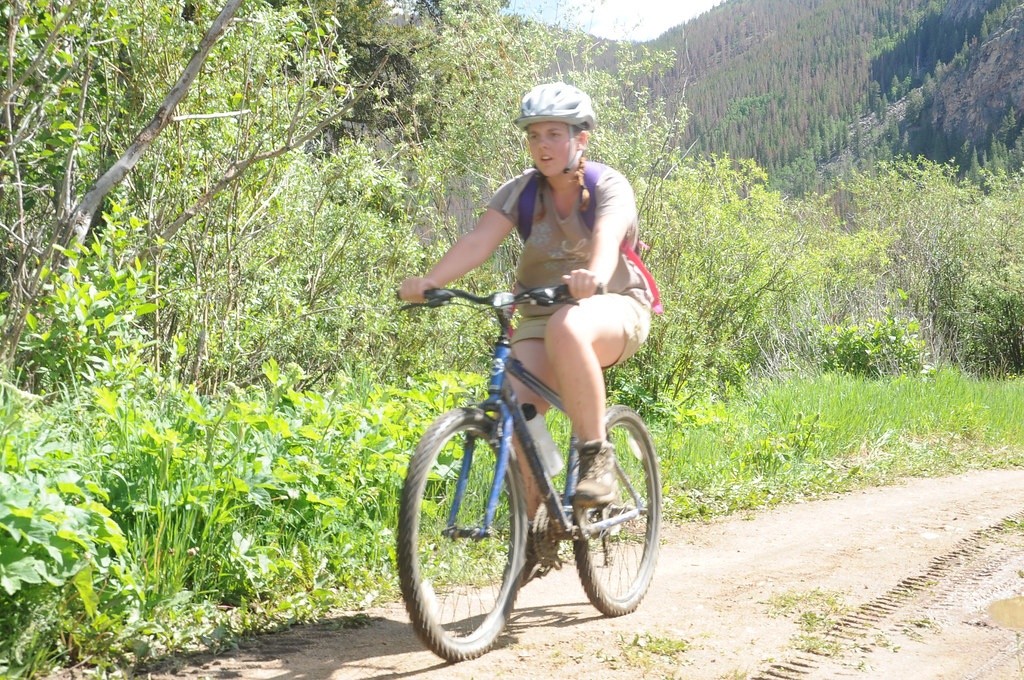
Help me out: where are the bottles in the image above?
[522,403,565,479]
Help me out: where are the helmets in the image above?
[514,81,595,131]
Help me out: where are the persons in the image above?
[400,81,661,586]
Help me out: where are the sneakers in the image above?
[574,438,620,505]
[504,546,543,588]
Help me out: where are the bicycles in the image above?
[391,279,663,661]
